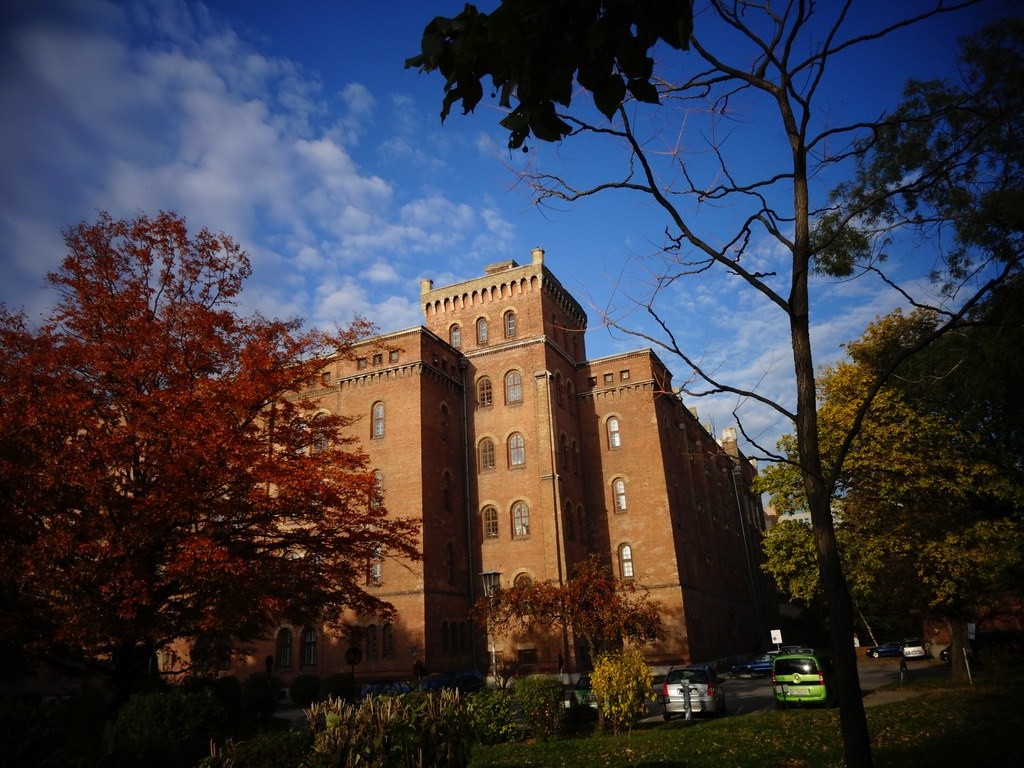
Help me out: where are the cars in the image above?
[731,655,774,679]
[660,665,726,719]
[772,655,832,706]
[865,641,904,658]
[561,672,602,720]
[901,638,925,658]
